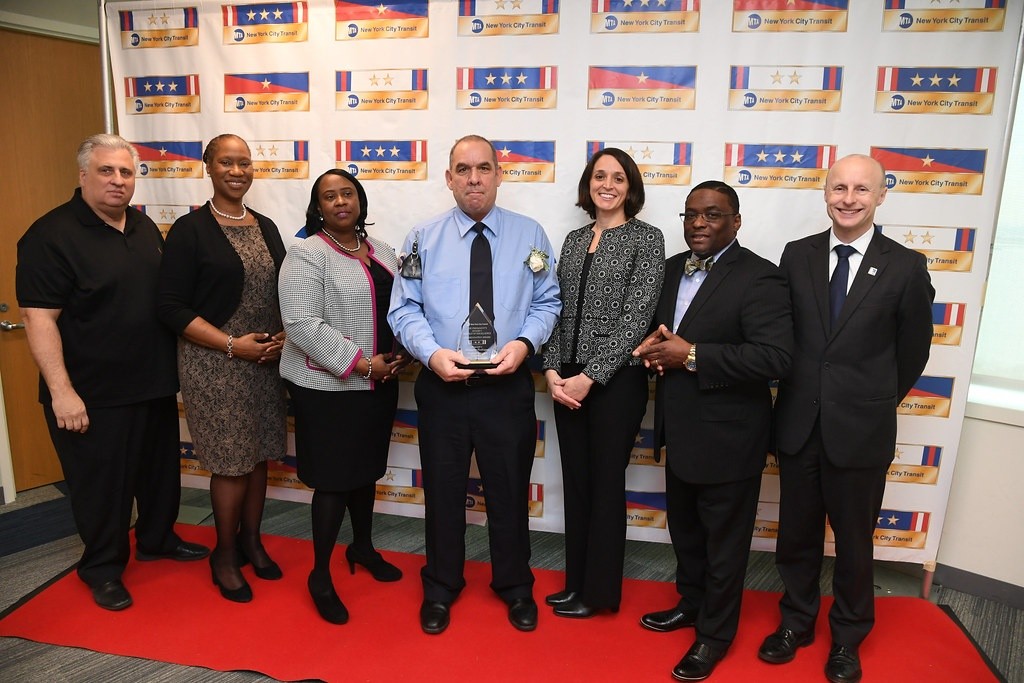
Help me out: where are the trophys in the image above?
[455,302,497,370]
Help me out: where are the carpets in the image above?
[0,523,1007,683]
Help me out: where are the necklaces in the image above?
[322,228,360,252]
[210,198,247,219]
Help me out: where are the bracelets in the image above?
[226,335,233,358]
[360,356,372,380]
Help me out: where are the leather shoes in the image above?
[823,641,862,683]
[640,606,695,632]
[420,595,450,634]
[494,586,538,631]
[135,536,209,560]
[77,563,132,611]
[672,639,727,680]
[758,624,815,663]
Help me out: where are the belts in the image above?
[457,363,498,380]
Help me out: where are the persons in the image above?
[278,169,419,624]
[540,148,665,618]
[159,134,287,602]
[632,181,794,680]
[387,135,562,634]
[15,133,211,610]
[758,154,936,683]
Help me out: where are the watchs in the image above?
[682,344,696,372]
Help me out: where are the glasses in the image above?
[679,211,736,224]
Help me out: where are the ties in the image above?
[468,221,497,353]
[828,244,858,335]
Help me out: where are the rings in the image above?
[656,360,659,366]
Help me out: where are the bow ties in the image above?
[685,255,715,277]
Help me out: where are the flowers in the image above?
[523,243,550,272]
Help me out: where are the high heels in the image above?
[345,543,403,582]
[241,541,283,580]
[546,587,583,606]
[553,596,619,618]
[307,569,349,623]
[209,548,253,602]
